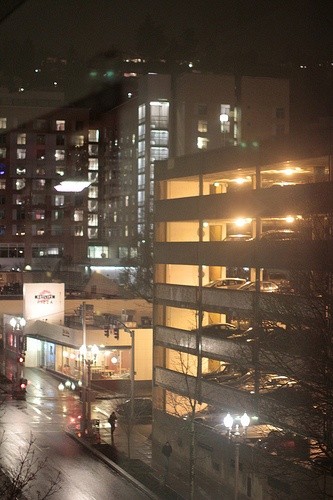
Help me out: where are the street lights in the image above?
[79,344,100,441]
[9,318,26,392]
[223,412,251,500]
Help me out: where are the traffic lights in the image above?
[114,327,119,340]
[104,325,110,338]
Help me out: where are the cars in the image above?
[261,229,301,240]
[115,397,153,418]
[201,362,304,395]
[181,404,268,432]
[237,280,281,293]
[190,323,245,339]
[223,234,253,241]
[202,278,246,290]
[257,428,312,460]
[226,322,291,344]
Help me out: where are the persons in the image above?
[107,410,119,442]
[162,441,172,467]
